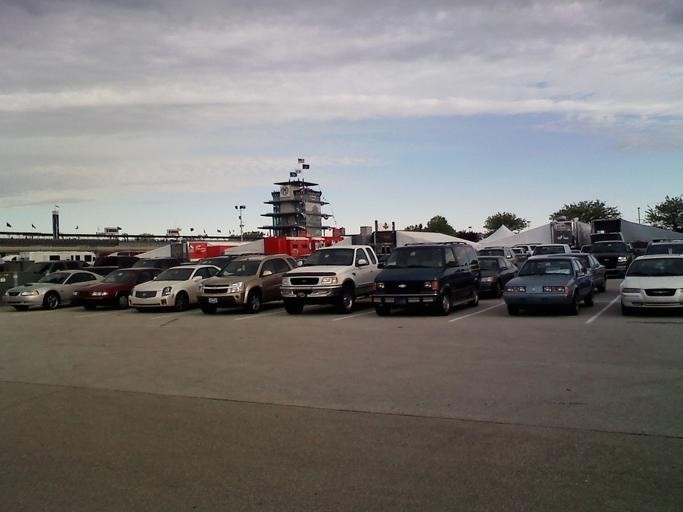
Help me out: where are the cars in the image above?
[280,244,386,315]
[0,260,106,311]
[128,264,222,312]
[477,239,634,317]
[619,238,683,317]
[93,255,232,268]
[72,268,164,310]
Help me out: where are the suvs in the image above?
[197,251,298,315]
[370,241,480,318]
